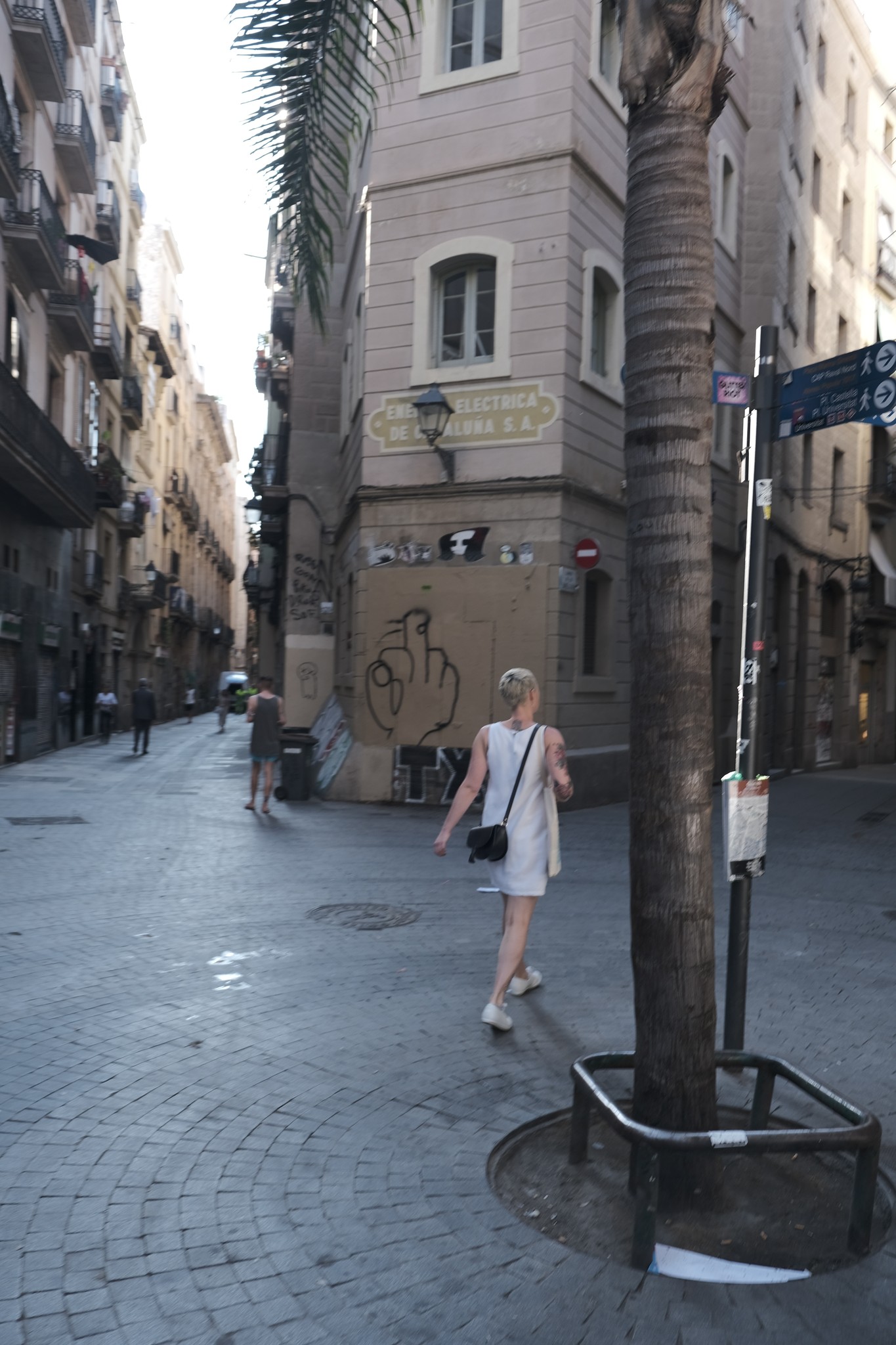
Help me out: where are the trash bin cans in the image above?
[277,727,312,733]
[274,733,319,802]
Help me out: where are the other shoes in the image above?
[481,1003,513,1031]
[505,966,542,996]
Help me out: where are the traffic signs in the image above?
[774,340,896,440]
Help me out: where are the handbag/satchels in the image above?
[465,823,508,863]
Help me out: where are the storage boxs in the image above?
[321,742,392,801]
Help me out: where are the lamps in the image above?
[122,560,159,595]
[816,553,875,594]
[245,494,339,546]
[411,382,456,486]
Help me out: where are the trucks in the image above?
[217,671,248,710]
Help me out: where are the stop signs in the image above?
[575,538,598,569]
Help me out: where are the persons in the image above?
[243,555,254,579]
[130,678,156,754]
[235,683,258,715]
[216,689,229,734]
[145,560,155,571]
[244,676,287,812]
[434,667,573,1030]
[183,682,196,723]
[96,683,119,744]
